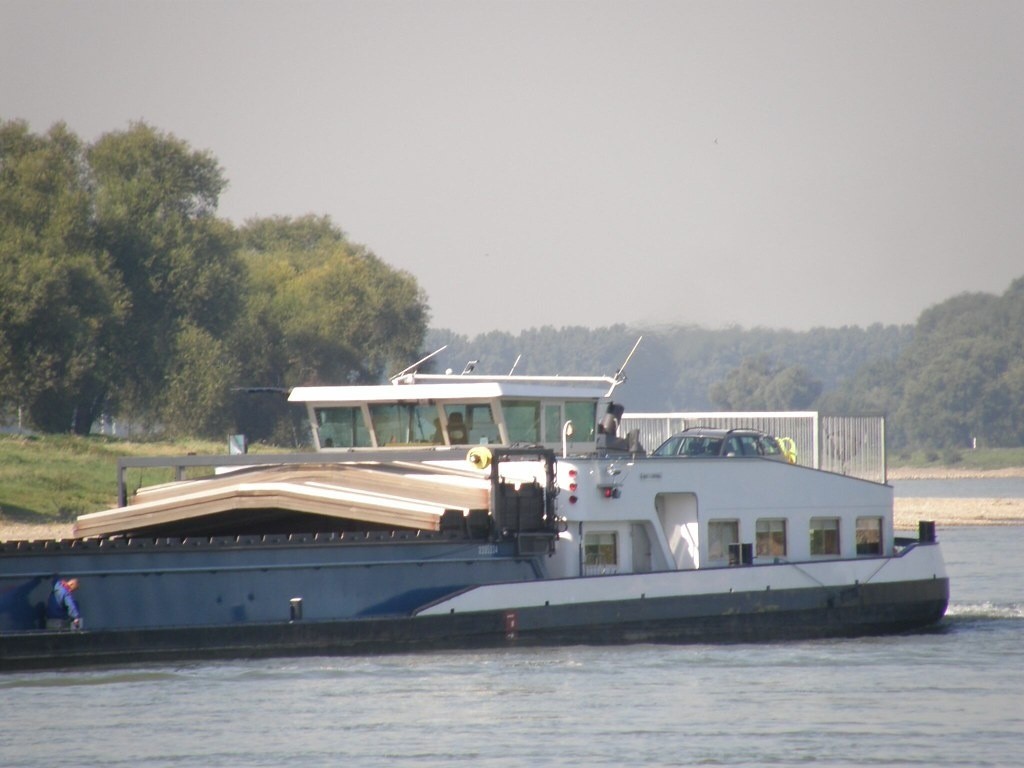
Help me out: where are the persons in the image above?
[45,578,81,631]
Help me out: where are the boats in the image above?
[1,333,953,674]
